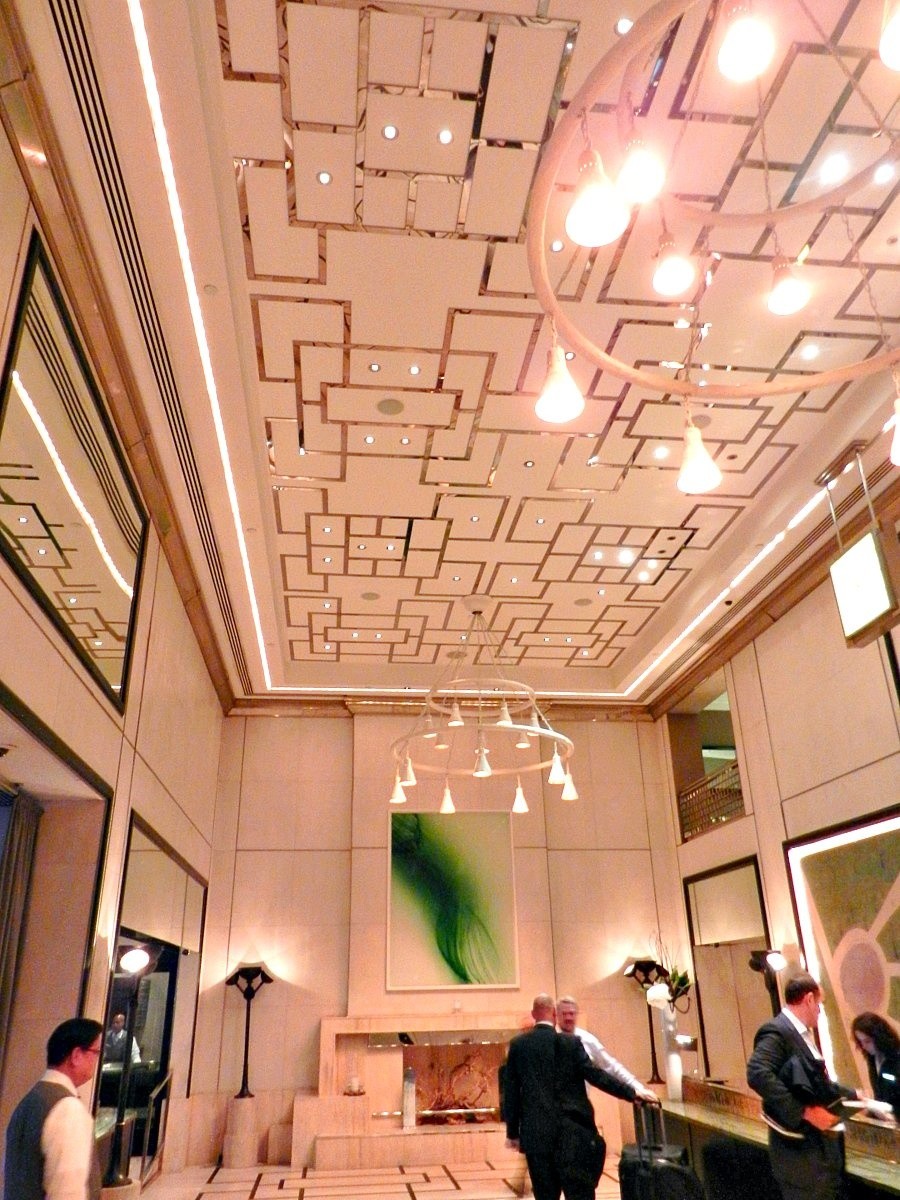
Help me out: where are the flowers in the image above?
[638,965,691,1014]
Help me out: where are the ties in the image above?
[112,1033,119,1045]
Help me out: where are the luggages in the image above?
[618,1100,705,1199]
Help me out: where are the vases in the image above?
[663,1008,682,1102]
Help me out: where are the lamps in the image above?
[749,950,787,1017]
[527,1,900,492]
[622,959,669,1083]
[225,966,274,1099]
[102,945,165,1187]
[389,594,579,814]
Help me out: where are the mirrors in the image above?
[0,224,153,716]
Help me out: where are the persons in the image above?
[4,1018,103,1200]
[554,996,658,1100]
[851,1011,900,1125]
[496,1017,535,1128]
[501,993,657,1200]
[103,1014,141,1063]
[746,971,863,1200]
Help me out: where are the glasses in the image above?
[86,1046,101,1056]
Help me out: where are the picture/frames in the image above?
[385,810,520,993]
